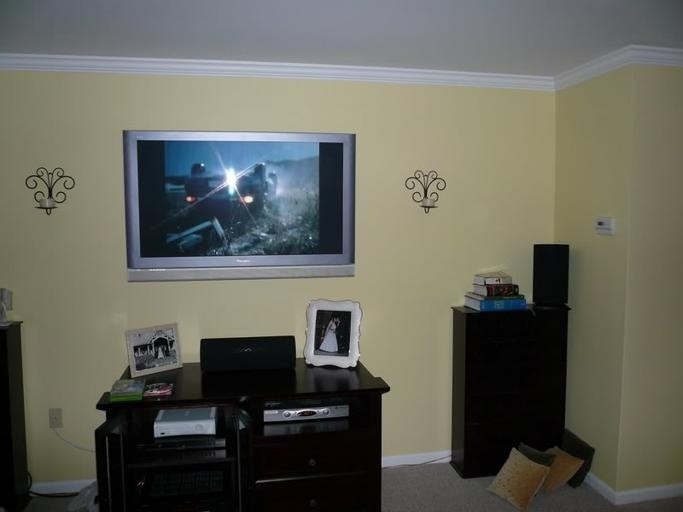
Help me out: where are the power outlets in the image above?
[49,408,63,428]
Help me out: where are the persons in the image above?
[333,315,349,353]
[317,317,337,352]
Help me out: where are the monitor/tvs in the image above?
[122,129,356,282]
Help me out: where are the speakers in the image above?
[200,335,296,372]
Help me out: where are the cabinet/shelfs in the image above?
[94,395,383,512]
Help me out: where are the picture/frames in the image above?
[303,299,362,367]
[124,322,184,378]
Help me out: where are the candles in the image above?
[423,199,434,206]
[40,198,54,207]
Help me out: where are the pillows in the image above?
[485,425,596,512]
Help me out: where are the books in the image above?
[462,296,525,311]
[472,270,511,286]
[143,383,173,396]
[472,284,519,296]
[109,379,145,400]
[464,292,524,301]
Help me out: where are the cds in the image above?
[146,382,173,394]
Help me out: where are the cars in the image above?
[183,163,278,218]
